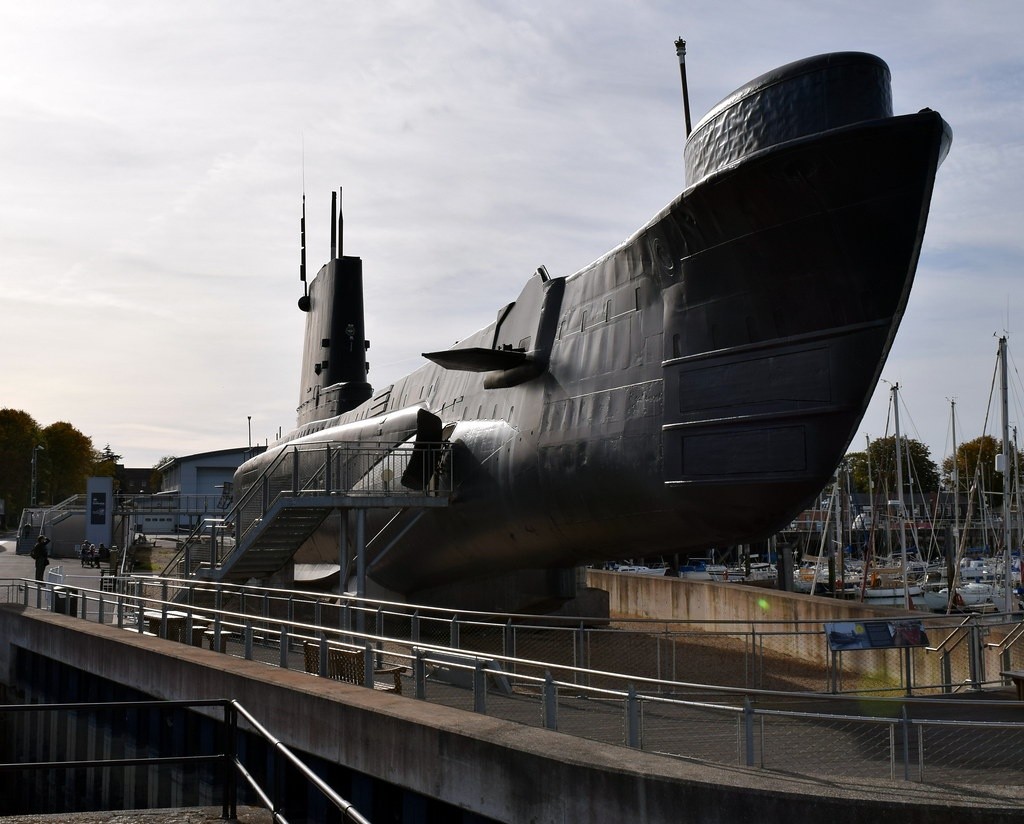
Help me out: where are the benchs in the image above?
[78,549,98,565]
[303,641,407,695]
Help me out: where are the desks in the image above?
[134,611,185,642]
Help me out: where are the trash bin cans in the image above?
[52,585,78,620]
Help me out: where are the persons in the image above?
[35,535,51,584]
[95,543,110,568]
[24,522,31,539]
[80,540,96,568]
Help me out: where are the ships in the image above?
[152,37,951,625]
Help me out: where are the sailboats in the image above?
[599,327,1024,616]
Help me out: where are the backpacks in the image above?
[30,546,38,559]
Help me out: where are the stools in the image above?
[204,630,232,653]
[192,625,207,648]
